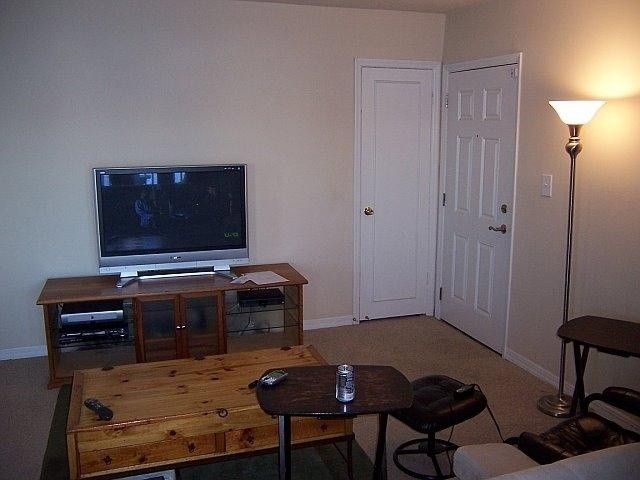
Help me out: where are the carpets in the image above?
[41,386,375,479]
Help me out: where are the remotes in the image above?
[83,398,113,421]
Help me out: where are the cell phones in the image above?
[261,370,288,386]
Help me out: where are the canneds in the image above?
[335,364,356,403]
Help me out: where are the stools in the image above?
[390,376,486,479]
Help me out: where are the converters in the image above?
[453,385,473,400]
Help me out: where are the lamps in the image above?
[536,99,608,416]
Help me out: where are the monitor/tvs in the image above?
[92,163,250,287]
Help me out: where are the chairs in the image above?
[519,387,640,466]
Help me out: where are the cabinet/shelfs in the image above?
[37,263,308,389]
